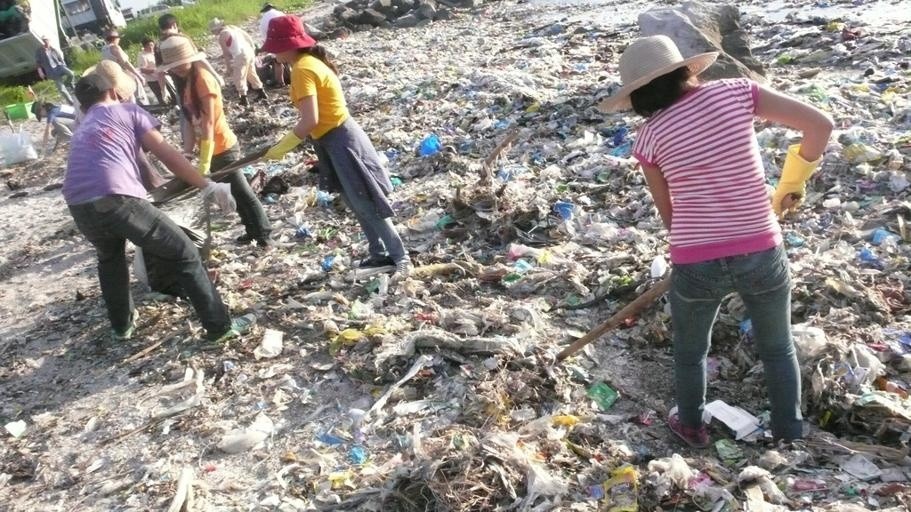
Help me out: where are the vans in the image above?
[122,0,196,23]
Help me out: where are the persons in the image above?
[258,16,416,285]
[103,30,153,107]
[35,35,78,107]
[32,101,78,153]
[259,1,288,90]
[62,72,257,347]
[138,39,176,106]
[595,36,836,449]
[157,35,275,253]
[209,18,270,111]
[73,59,162,191]
[154,13,227,162]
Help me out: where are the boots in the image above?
[239,94,250,106]
[254,88,268,102]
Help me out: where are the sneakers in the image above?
[207,313,257,344]
[115,308,140,341]
[237,234,254,244]
[667,406,709,448]
[352,254,391,267]
[257,238,275,249]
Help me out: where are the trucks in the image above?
[0,0,129,84]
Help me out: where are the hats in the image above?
[207,17,225,32]
[107,29,126,41]
[259,14,316,53]
[260,2,273,12]
[156,35,207,72]
[83,59,138,101]
[598,34,719,114]
[31,98,43,122]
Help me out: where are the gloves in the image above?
[263,128,306,161]
[197,139,216,176]
[772,144,823,219]
